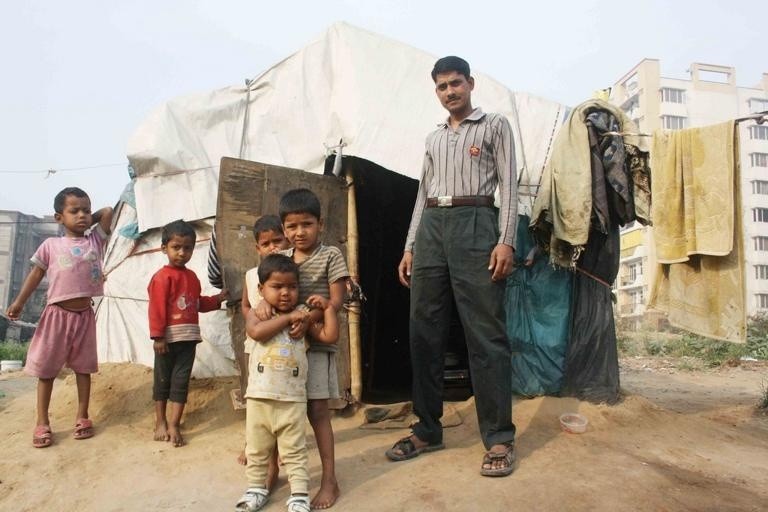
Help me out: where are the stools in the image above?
[423,194,494,209]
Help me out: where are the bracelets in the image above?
[559,413,588,433]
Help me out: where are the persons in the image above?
[237,217,295,467]
[7,187,112,448]
[257,189,351,507]
[235,253,338,512]
[147,220,229,448]
[384,56,518,477]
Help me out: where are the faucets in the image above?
[75,416,97,439]
[480,440,516,477]
[234,486,272,512]
[283,494,312,512]
[31,426,53,448]
[383,433,446,462]
